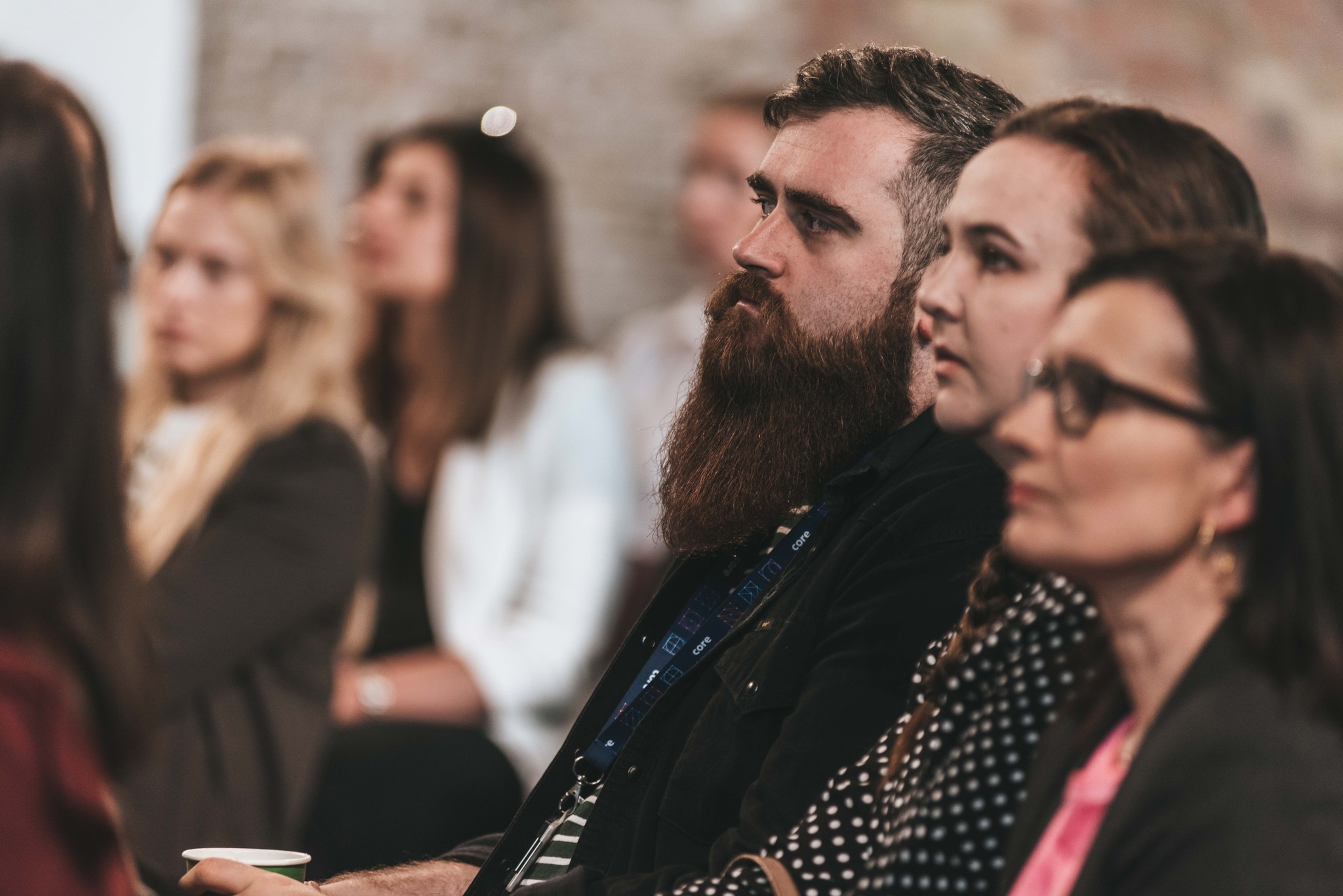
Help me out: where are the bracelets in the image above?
[355,661,392,720]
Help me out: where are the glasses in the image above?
[1021,358,1220,438]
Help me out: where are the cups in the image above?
[183,848,312,896]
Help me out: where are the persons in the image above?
[657,94,1267,896]
[0,60,786,896]
[991,234,1343,896]
[180,45,1027,896]
